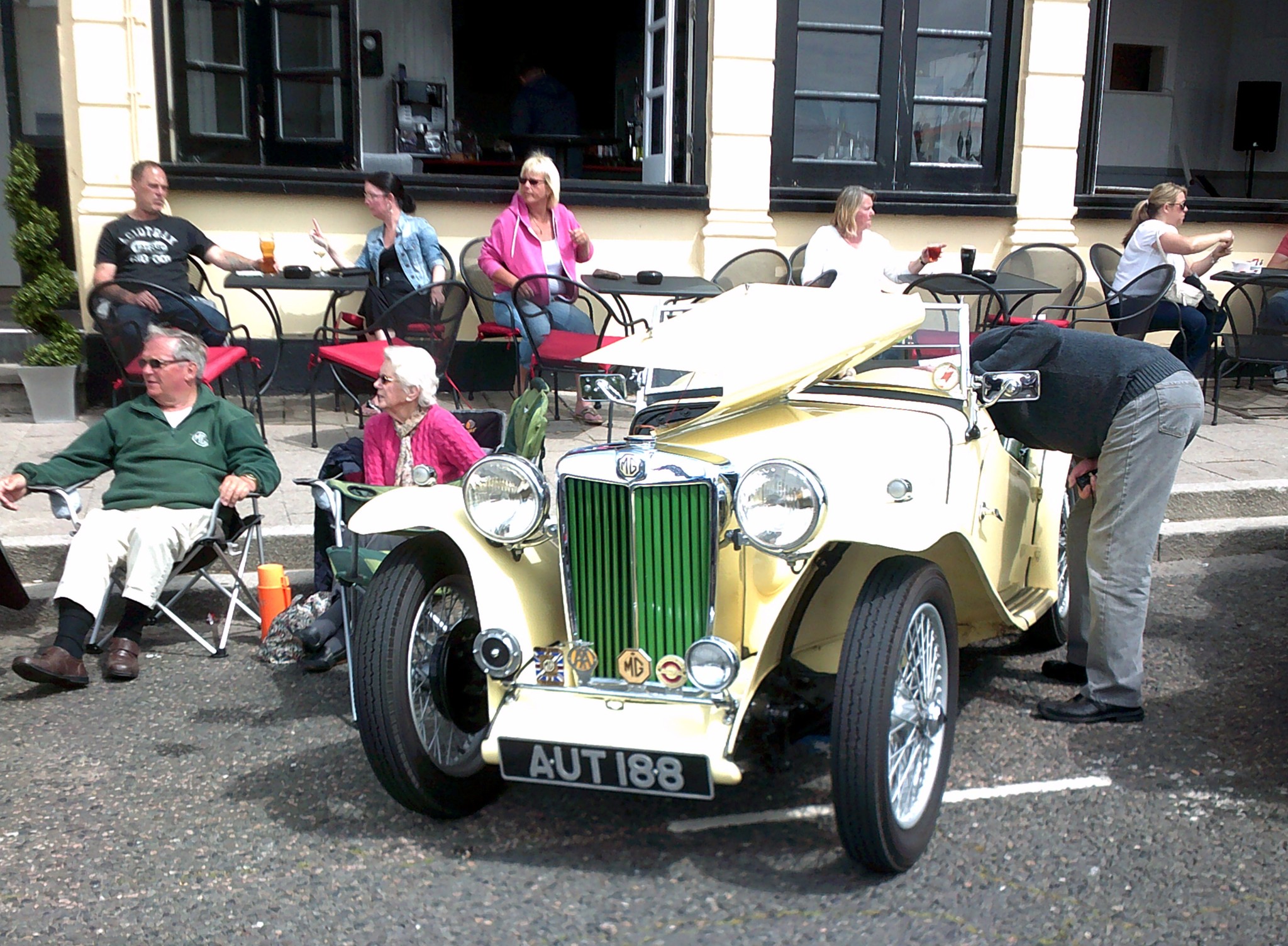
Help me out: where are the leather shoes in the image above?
[296,623,324,650]
[11,646,88,687]
[299,646,347,669]
[1041,659,1089,685]
[105,636,141,680]
[1037,693,1144,722]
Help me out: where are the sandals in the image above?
[354,398,380,414]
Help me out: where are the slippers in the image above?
[576,404,604,425]
[514,383,529,398]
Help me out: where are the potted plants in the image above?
[2,139,84,424]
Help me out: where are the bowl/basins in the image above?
[283,266,312,279]
[972,269,998,284]
[636,270,663,285]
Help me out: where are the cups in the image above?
[961,247,977,275]
[927,242,941,261]
[259,235,275,273]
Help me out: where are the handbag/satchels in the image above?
[260,591,340,668]
[1165,279,1203,307]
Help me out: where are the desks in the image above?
[581,275,723,410]
[898,272,1062,332]
[1210,267,1288,401]
[224,270,370,428]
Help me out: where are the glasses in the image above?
[364,192,387,198]
[1157,200,1186,210]
[137,357,198,371]
[377,372,395,383]
[520,177,548,186]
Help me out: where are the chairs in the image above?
[326,379,553,713]
[84,279,268,445]
[788,242,807,286]
[310,279,469,447]
[1090,241,1195,376]
[975,243,1087,330]
[683,248,792,313]
[28,477,265,658]
[323,244,457,413]
[1203,274,1288,424]
[459,236,601,409]
[294,408,508,590]
[109,256,248,409]
[512,273,652,444]
[1034,263,1176,340]
[900,273,1006,358]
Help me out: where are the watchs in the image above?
[243,474,257,490]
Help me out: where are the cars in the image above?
[346,283,1097,874]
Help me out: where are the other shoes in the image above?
[1272,369,1288,390]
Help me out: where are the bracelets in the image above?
[920,256,926,265]
[1210,253,1215,261]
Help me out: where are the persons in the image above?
[310,170,447,414]
[93,161,280,400]
[477,156,604,423]
[970,321,1204,722]
[1259,233,1288,393]
[802,186,946,359]
[0,328,282,688]
[1109,183,1234,375]
[291,345,486,670]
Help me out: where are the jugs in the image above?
[257,564,292,642]
